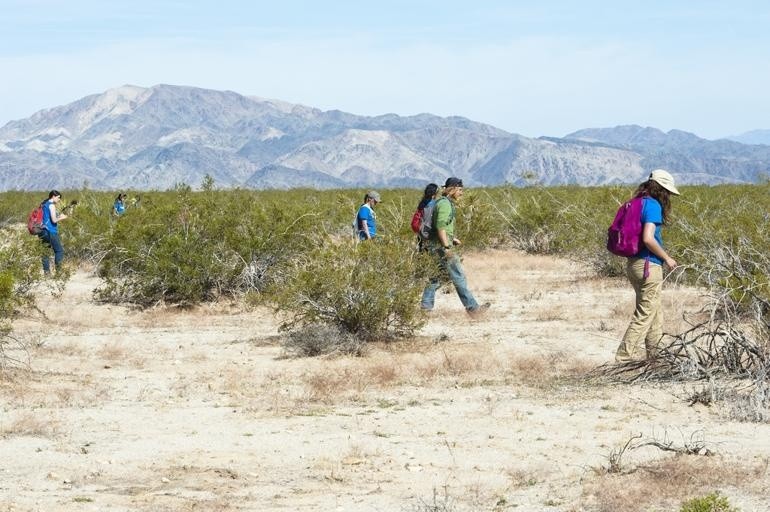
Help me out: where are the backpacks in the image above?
[607,194,652,257]
[28,202,53,235]
[412,198,454,240]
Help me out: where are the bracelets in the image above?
[444,245,452,249]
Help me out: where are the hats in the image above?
[649,169,681,196]
[368,191,382,203]
[441,178,463,187]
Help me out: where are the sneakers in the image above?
[467,303,491,320]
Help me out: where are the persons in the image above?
[615,170,682,364]
[357,190,382,241]
[39,190,68,279]
[421,176,491,319]
[62,200,77,215]
[112,194,126,217]
[418,184,439,253]
